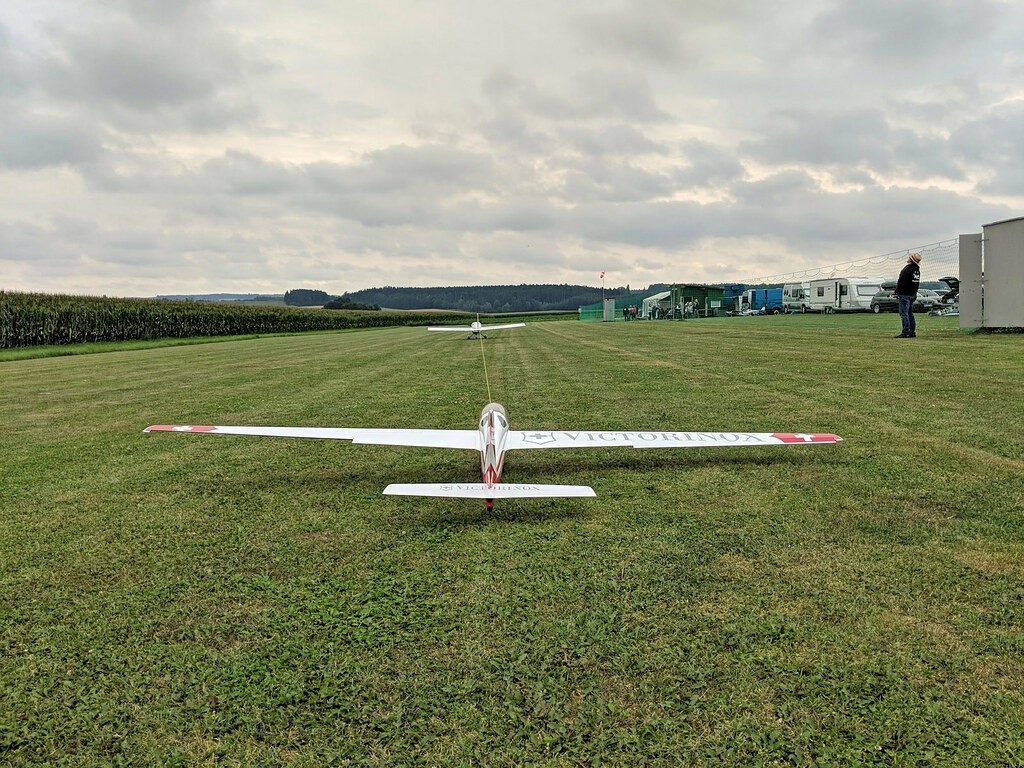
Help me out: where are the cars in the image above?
[870,278,960,312]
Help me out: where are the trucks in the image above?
[782,278,882,313]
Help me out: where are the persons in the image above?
[892,252,922,338]
[623,305,665,322]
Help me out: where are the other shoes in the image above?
[894,333,909,338]
[908,332,916,337]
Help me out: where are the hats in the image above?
[908,253,922,268]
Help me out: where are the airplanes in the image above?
[144,403,844,509]
[427,313,526,340]
